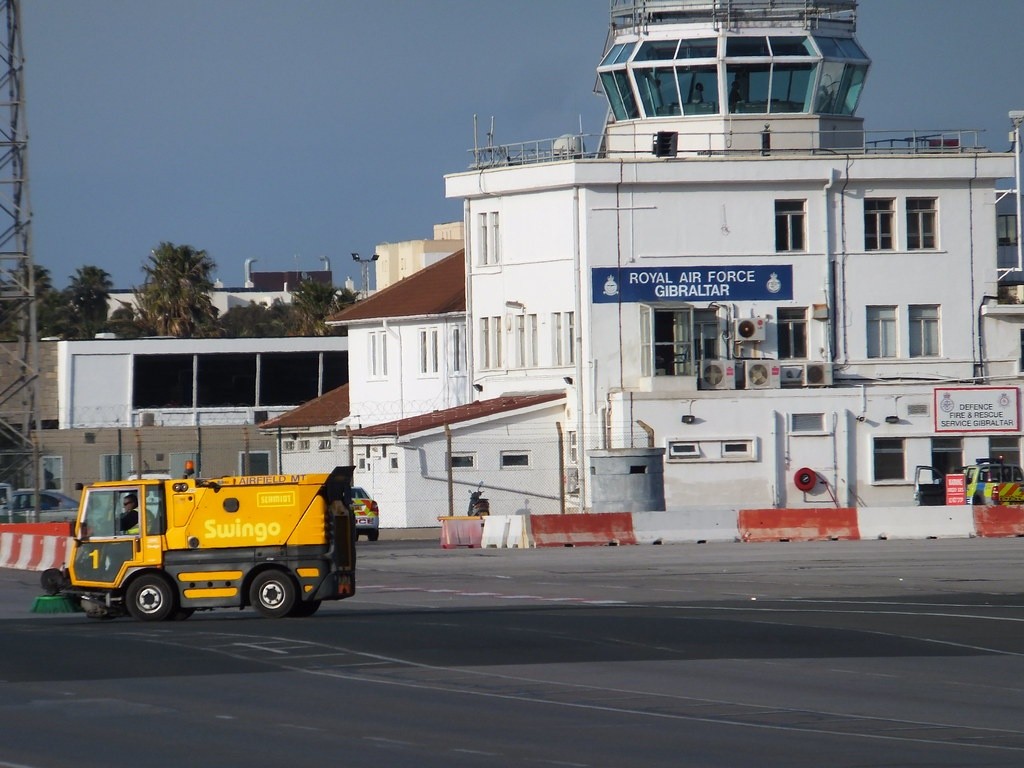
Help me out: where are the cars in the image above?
[0,490,80,524]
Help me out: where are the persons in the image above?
[690,82,703,104]
[119,494,155,535]
[728,81,742,113]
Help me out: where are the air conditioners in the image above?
[742,360,781,390]
[801,362,833,386]
[698,360,736,390]
[734,317,766,341]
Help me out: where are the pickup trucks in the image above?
[914,458,1024,506]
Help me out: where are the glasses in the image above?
[124,502,132,506]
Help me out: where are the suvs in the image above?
[351,486,379,542]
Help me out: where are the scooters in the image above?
[468,480,490,517]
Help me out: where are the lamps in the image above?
[563,377,573,385]
[885,416,899,423]
[472,384,483,391]
[682,415,695,424]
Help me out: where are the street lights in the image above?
[350,251,379,300]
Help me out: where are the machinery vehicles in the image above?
[29,461,358,621]
